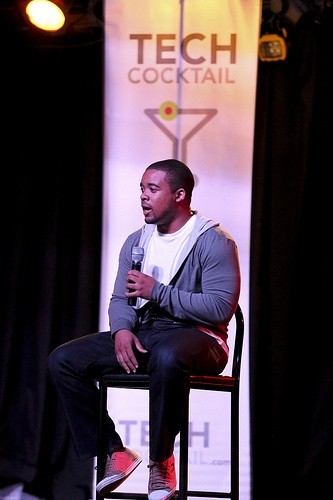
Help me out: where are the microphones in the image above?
[127,246,145,305]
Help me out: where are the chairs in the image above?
[96,301,244,500]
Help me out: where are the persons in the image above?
[48,159,240,500]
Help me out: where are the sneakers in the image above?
[95,447,143,495]
[147,453,176,499]
